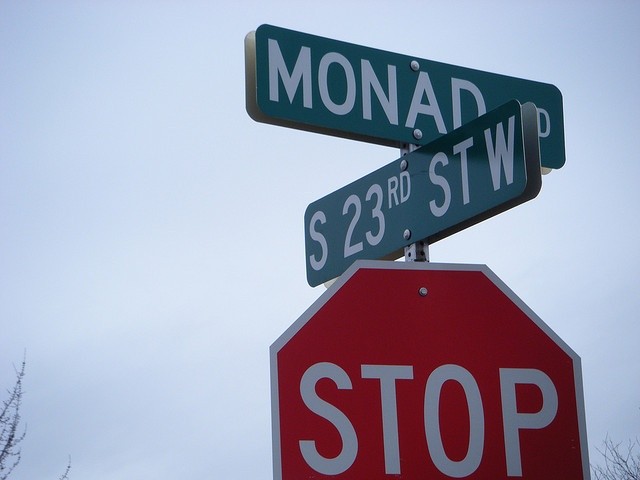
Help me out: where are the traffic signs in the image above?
[304,98,541,288]
[244,23,566,174]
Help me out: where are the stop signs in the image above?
[269,259,590,479]
